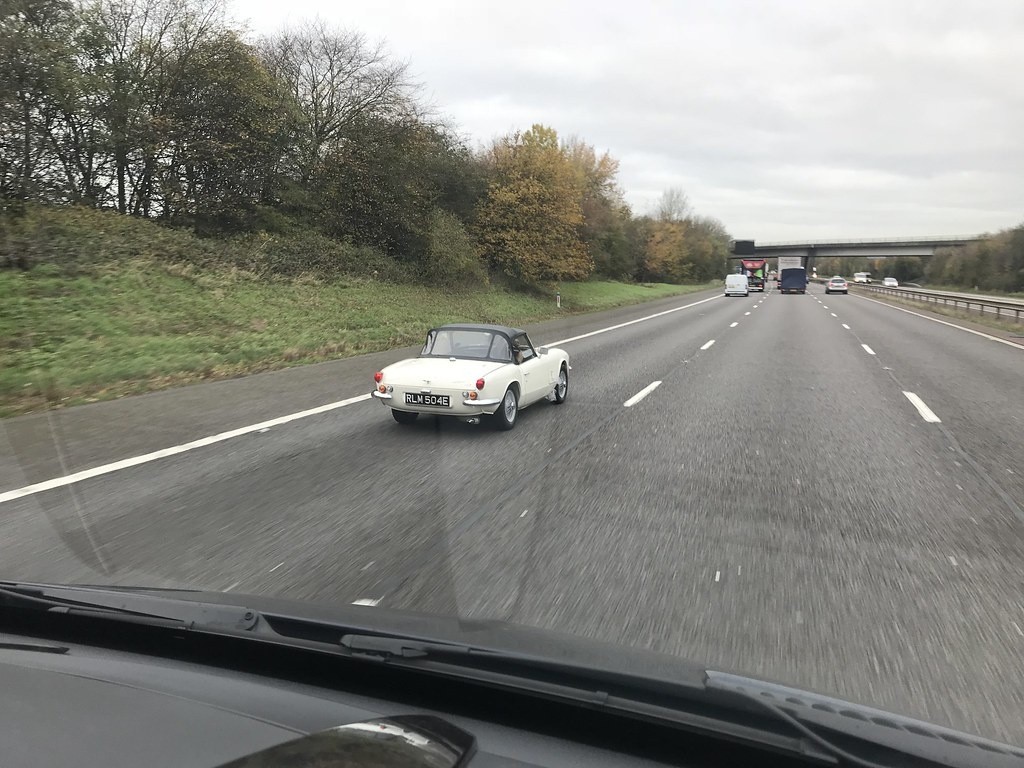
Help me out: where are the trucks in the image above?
[853,272,871,284]
[780,268,806,294]
[740,259,767,292]
[724,274,749,297]
[777,257,801,289]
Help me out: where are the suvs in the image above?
[825,275,848,295]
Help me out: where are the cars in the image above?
[371,323,572,431]
[882,278,899,287]
